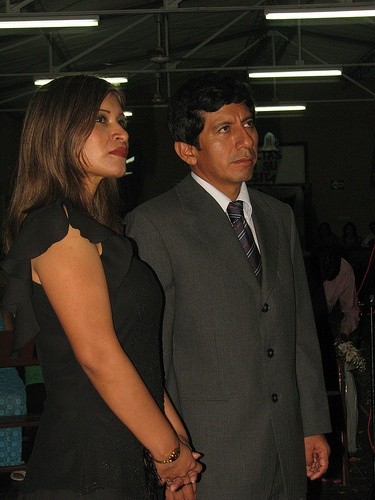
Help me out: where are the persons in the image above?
[0,75,205,500]
[124,79,331,500]
[304,221,375,490]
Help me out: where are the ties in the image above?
[226,200,263,286]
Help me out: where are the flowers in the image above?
[333,337,367,373]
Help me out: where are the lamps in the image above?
[33,74,128,86]
[247,68,343,78]
[264,6,375,20]
[0,15,99,28]
[254,103,307,111]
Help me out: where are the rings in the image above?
[166,477,176,483]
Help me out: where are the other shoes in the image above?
[10,470,26,481]
[322,479,342,486]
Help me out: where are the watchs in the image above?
[151,439,181,464]
